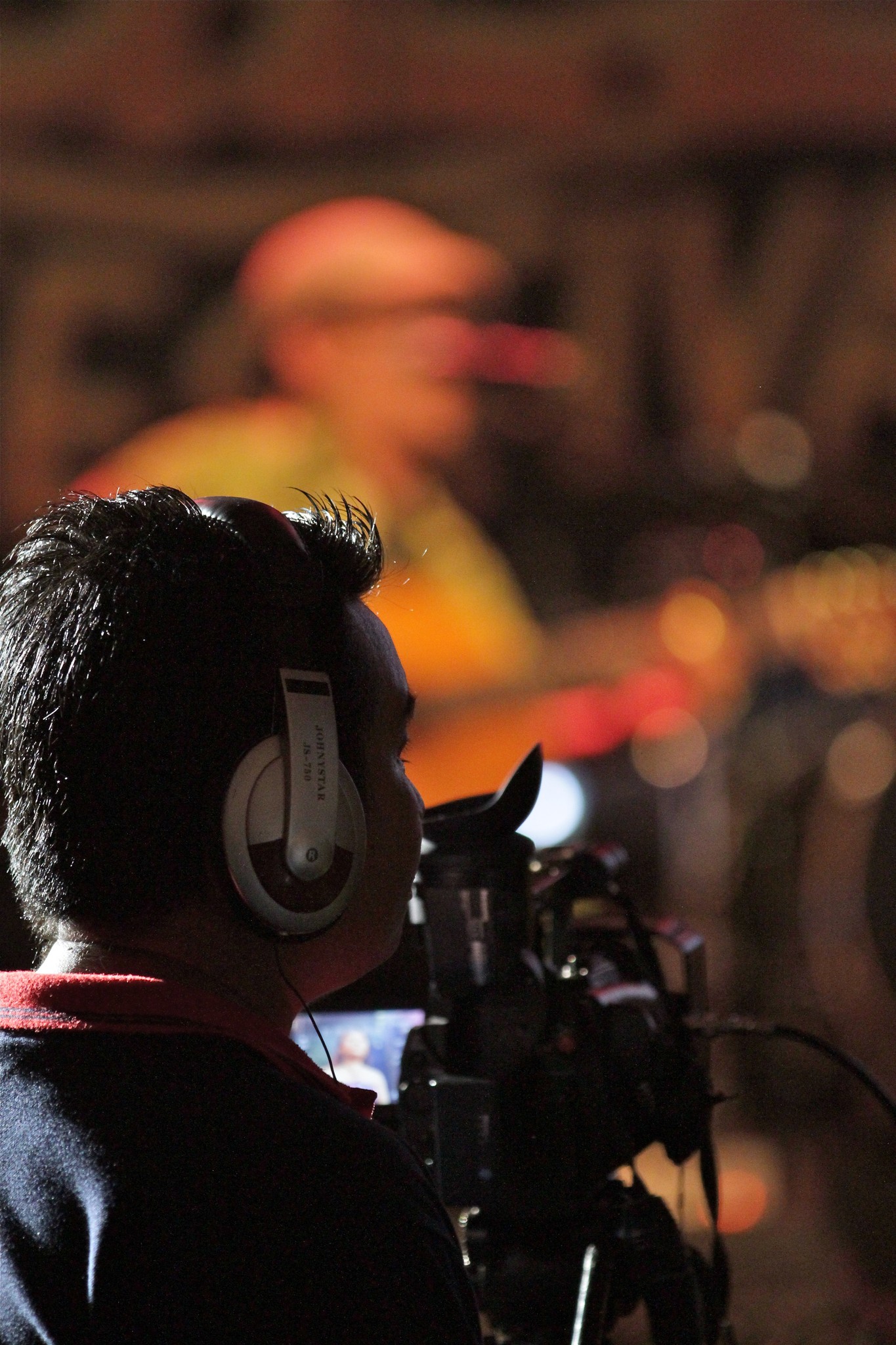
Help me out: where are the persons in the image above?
[1,194,750,1345]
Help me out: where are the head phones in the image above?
[179,497,371,941]
